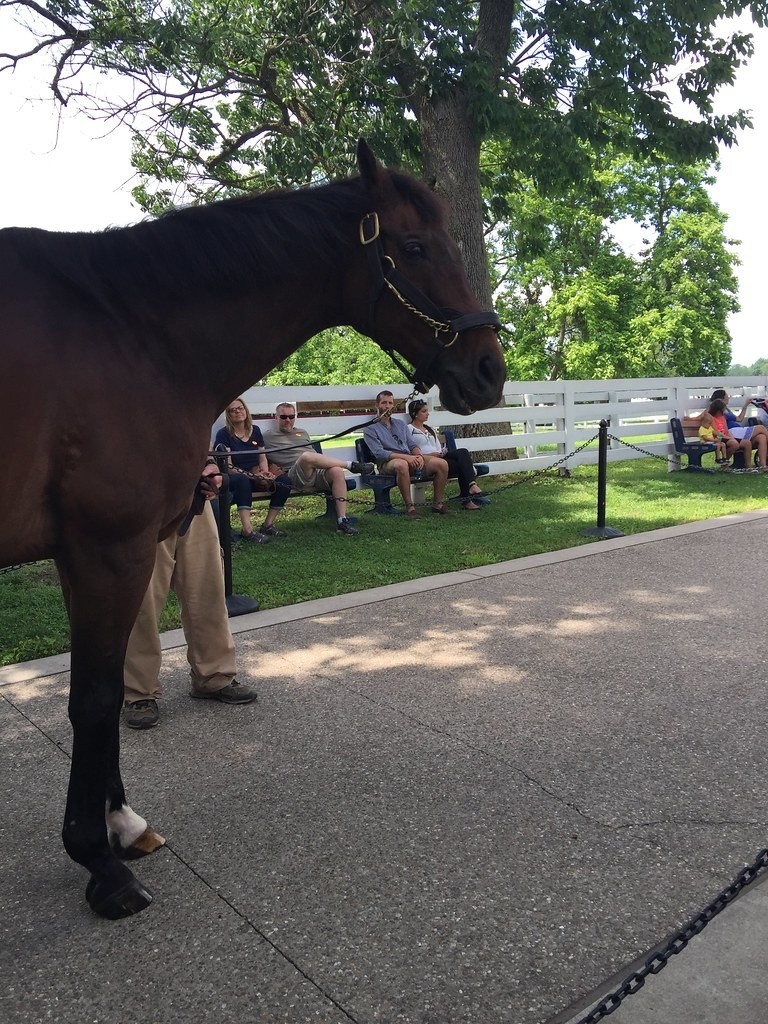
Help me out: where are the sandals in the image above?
[467,483,481,495]
[462,500,479,509]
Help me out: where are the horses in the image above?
[1,140,508,917]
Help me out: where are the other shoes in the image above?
[715,459,722,463]
[405,510,421,521]
[722,458,729,463]
[432,505,457,515]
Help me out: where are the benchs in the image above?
[670,414,757,470]
[206,441,356,543]
[356,429,489,514]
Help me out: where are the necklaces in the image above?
[234,431,246,439]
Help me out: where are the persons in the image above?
[683,390,768,473]
[124,454,258,729]
[363,390,452,518]
[697,415,730,463]
[260,403,375,536]
[708,401,739,472]
[406,399,492,511]
[214,398,291,543]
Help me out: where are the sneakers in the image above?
[260,523,287,538]
[336,518,359,536]
[189,678,257,704]
[241,529,268,543]
[350,462,374,477]
[124,699,159,728]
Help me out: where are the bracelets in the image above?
[206,459,218,466]
[743,405,747,409]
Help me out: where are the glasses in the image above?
[280,414,295,420]
[414,399,423,409]
[229,406,245,413]
